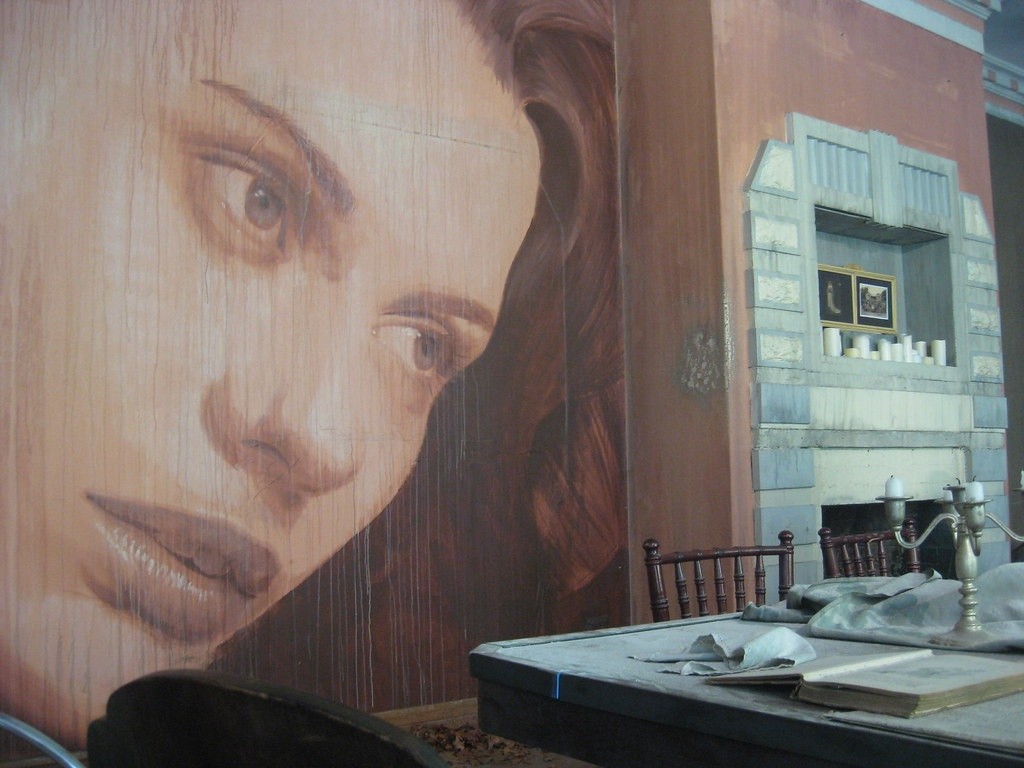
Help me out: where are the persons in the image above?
[0,2,626,756]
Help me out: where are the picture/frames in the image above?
[818,264,854,330]
[854,270,896,334]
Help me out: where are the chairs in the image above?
[817,519,921,578]
[640,530,794,620]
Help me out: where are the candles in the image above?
[961,476,985,502]
[885,474,906,498]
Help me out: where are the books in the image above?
[707,649,1023,718]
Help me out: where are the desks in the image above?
[469,612,1022,768]
